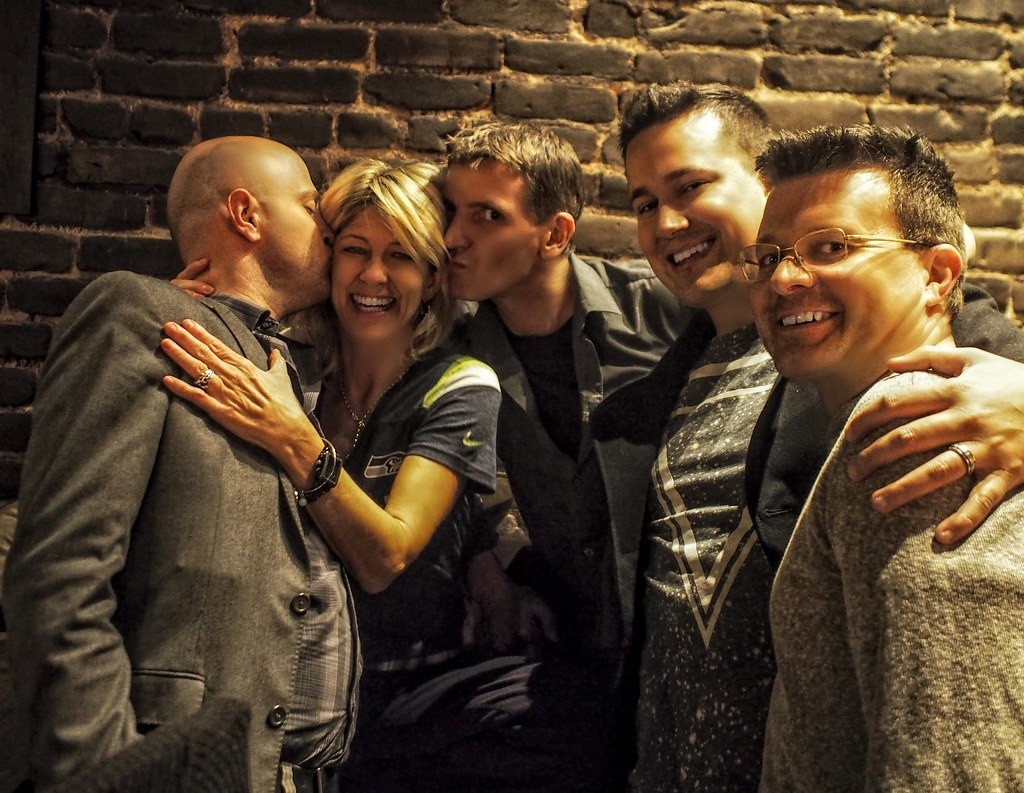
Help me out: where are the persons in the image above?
[736,123,1024,793]
[441,122,706,792]
[0,135,503,793]
[589,79,1024,793]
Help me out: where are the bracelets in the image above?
[298,436,337,506]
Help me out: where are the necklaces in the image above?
[337,366,410,460]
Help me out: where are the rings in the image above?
[195,369,214,389]
[949,442,976,475]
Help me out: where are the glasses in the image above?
[735,226,939,283]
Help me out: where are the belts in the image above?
[276,760,339,793]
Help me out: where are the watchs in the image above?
[299,443,342,503]
